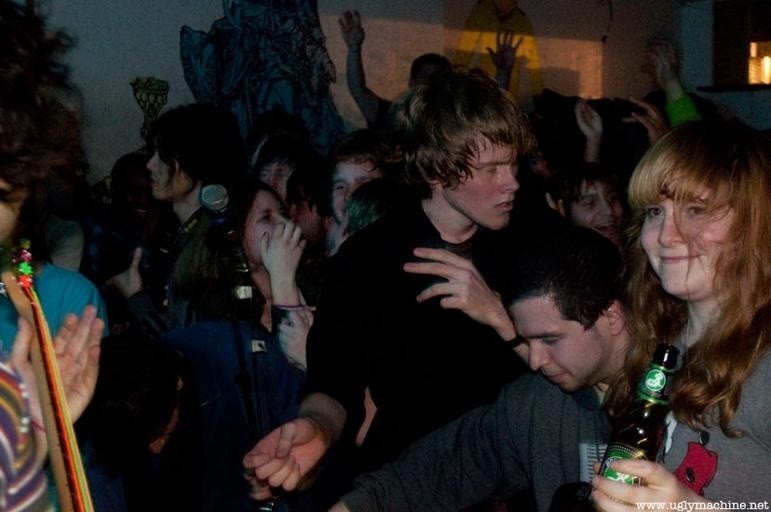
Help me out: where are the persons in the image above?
[47,96,397,512]
[585,116,771,510]
[1,0,110,512]
[638,34,703,130]
[327,239,642,511]
[0,193,109,367]
[338,8,527,142]
[556,160,629,255]
[619,94,666,146]
[242,68,624,511]
[573,96,606,166]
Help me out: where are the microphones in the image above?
[199,184,252,283]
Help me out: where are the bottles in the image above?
[584,339,682,511]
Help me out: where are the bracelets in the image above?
[503,335,525,352]
